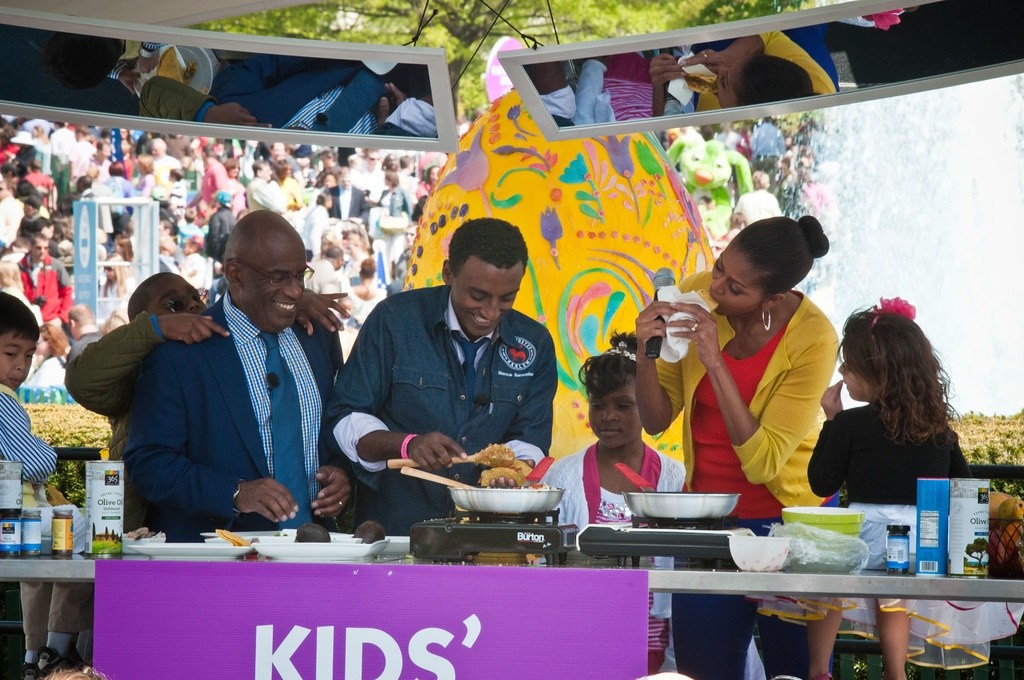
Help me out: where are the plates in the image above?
[375,536,413,555]
[251,535,393,563]
[126,542,254,559]
[121,536,166,554]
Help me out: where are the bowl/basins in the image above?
[781,506,865,539]
[725,534,791,571]
[615,463,742,520]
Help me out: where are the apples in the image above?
[988,491,1024,566]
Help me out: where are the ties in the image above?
[452,330,488,414]
[258,330,313,530]
[314,70,392,133]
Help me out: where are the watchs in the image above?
[234,478,247,514]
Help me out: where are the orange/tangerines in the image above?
[1003,521,1022,547]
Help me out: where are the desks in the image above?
[0,549,1023,680]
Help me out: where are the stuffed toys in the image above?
[662,131,752,255]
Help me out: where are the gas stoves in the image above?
[409,514,580,565]
[574,522,770,567]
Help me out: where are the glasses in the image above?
[226,258,315,287]
[155,288,208,316]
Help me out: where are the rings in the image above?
[702,50,707,57]
[692,323,699,332]
[338,500,343,506]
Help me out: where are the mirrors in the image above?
[496,0,1024,143]
[0,6,459,154]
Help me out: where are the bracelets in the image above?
[401,434,418,459]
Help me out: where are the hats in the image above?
[216,191,233,209]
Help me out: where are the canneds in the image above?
[83,460,125,556]
[0,459,43,558]
[50,509,73,557]
[886,476,991,580]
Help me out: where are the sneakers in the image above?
[21,641,93,680]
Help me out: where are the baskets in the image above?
[988,517,1024,579]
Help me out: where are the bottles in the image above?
[20,509,43,557]
[51,508,75,558]
[0,459,24,516]
[0,514,22,558]
[886,524,911,574]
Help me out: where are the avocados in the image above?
[296,523,331,543]
[353,519,385,544]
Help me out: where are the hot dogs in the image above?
[480,458,533,488]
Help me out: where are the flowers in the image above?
[873,296,916,317]
[860,8,906,30]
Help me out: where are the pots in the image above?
[446,487,566,512]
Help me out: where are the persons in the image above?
[746,297,1024,680]
[713,116,837,236]
[524,0,1024,159]
[635,216,840,680]
[536,331,687,680]
[0,24,438,141]
[322,218,559,537]
[0,115,452,680]
